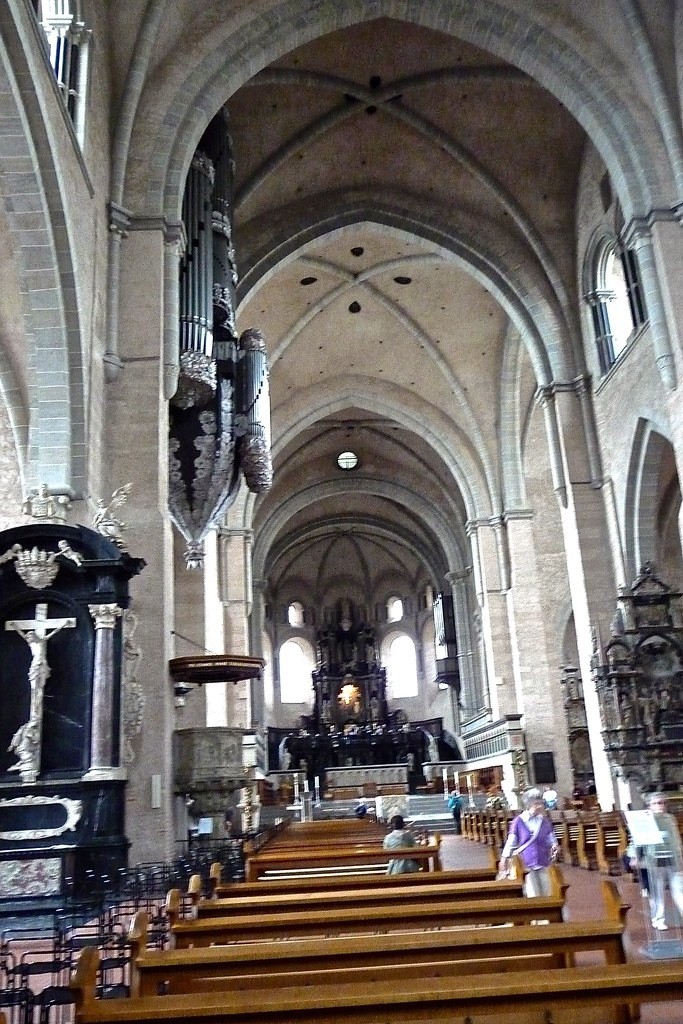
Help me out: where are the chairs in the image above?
[0,843,244,1024]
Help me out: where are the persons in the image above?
[8,620,72,719]
[0,544,28,564]
[543,786,557,810]
[56,540,84,567]
[572,784,584,801]
[629,792,683,930]
[91,499,125,528]
[384,815,419,874]
[448,791,464,835]
[587,780,596,794]
[354,800,366,817]
[498,790,568,925]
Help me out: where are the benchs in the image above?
[245,813,442,881]
[71,863,683,1024]
[457,803,683,884]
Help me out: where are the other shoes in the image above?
[651,920,668,931]
[642,888,648,897]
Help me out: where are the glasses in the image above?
[653,801,669,805]
[532,800,546,805]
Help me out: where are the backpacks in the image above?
[453,799,460,814]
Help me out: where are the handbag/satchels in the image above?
[498,855,524,885]
